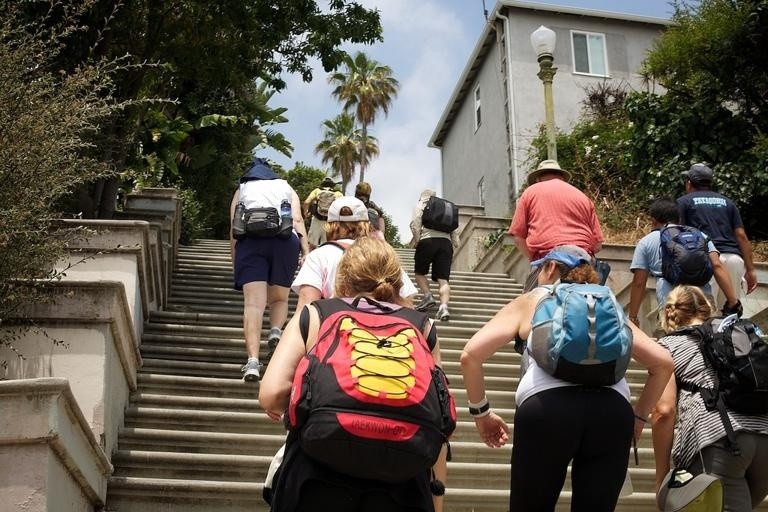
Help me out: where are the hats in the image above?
[529,160,570,185]
[327,197,369,223]
[531,245,591,268]
[682,163,713,185]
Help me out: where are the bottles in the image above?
[754,326,764,336]
[279,198,292,216]
[233,200,246,218]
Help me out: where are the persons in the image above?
[651,284,767,510]
[355,182,385,238]
[257,238,446,510]
[627,200,743,326]
[676,162,758,312]
[304,177,343,247]
[411,190,460,321]
[230,160,310,381]
[460,245,675,512]
[292,197,418,321]
[508,159,604,292]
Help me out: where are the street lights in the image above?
[530,23,560,165]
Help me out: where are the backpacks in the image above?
[666,314,768,456]
[286,296,456,482]
[311,192,382,231]
[514,283,632,385]
[652,225,713,286]
[422,196,458,232]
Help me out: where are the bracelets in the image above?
[628,315,641,322]
[468,396,492,420]
[636,414,649,429]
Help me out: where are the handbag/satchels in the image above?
[657,466,723,512]
[244,207,279,233]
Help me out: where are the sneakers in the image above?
[241,360,263,380]
[435,304,449,320]
[416,294,436,311]
[268,328,282,359]
